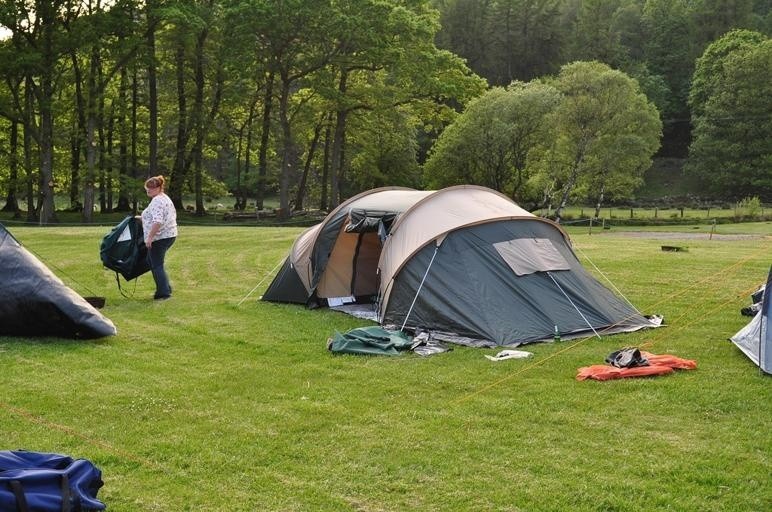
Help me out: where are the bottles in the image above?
[554,326,563,345]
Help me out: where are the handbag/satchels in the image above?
[100,216,151,281]
[0,451,106,512]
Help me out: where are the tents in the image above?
[238,185,670,348]
[0,221,117,341]
[726,264,772,378]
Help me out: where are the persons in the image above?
[134,175,177,300]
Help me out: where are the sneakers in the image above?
[153,286,172,300]
